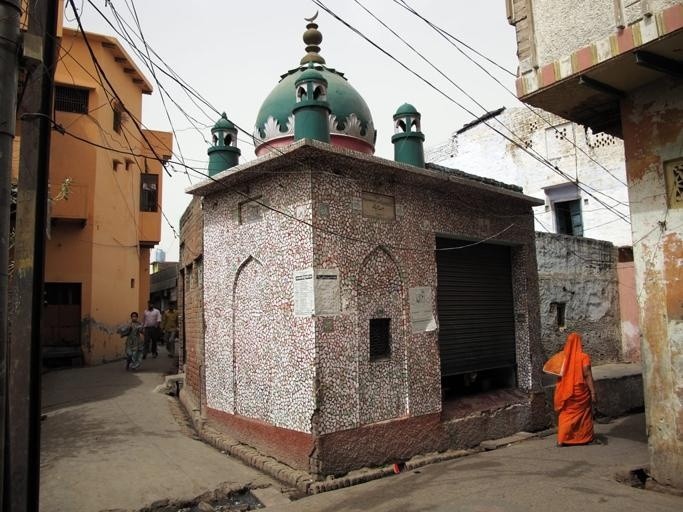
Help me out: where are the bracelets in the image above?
[589,391,597,395]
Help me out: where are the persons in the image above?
[139,299,161,360]
[159,300,179,359]
[117,311,148,372]
[542,329,601,447]
